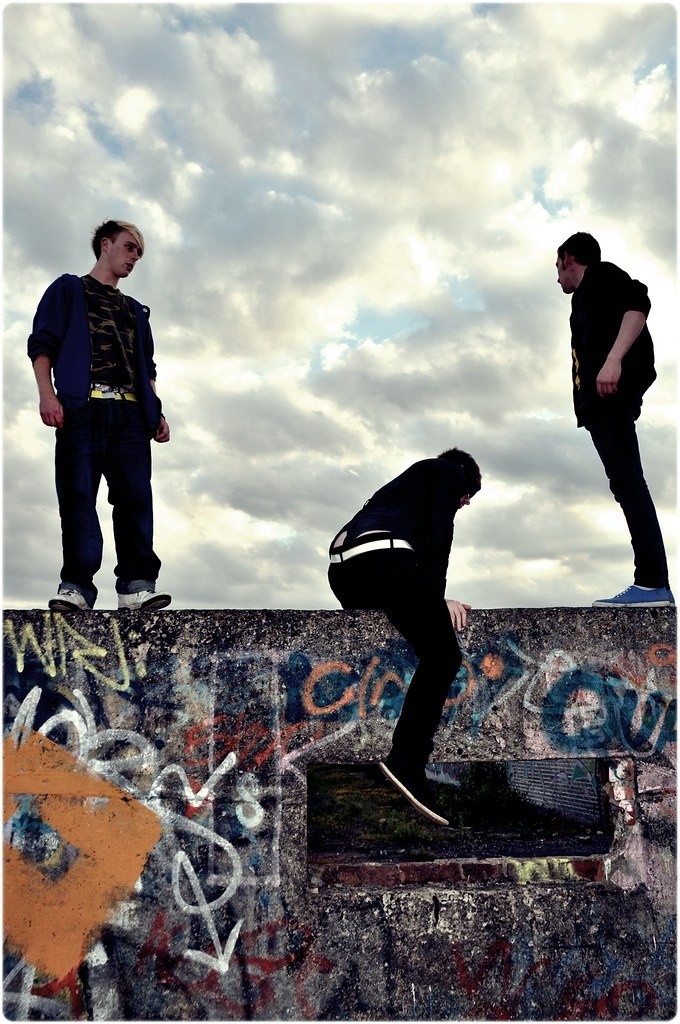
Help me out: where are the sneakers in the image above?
[594,585,669,607]
[376,754,449,828]
[665,587,676,608]
[116,590,172,611]
[49,590,94,609]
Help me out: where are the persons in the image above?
[326,445,481,824]
[553,231,675,609]
[21,220,169,615]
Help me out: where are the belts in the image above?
[91,390,137,402]
[329,539,412,564]
